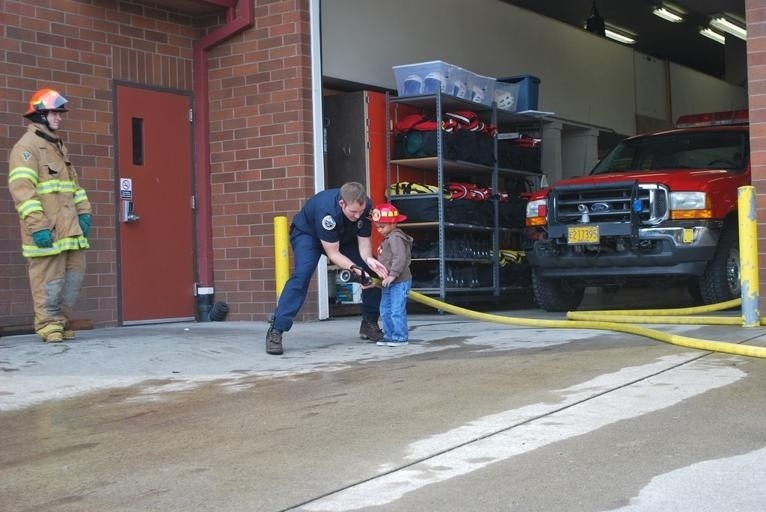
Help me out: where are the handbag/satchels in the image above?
[394,131,542,172]
[394,197,526,228]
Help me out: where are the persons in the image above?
[263,182,389,354]
[360,203,415,348]
[7,87,95,342]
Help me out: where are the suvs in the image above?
[524,110,749,315]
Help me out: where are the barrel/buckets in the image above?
[334,274,363,303]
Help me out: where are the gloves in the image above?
[79,213,92,238]
[32,229,54,249]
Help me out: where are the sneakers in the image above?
[358,319,384,341]
[376,339,392,346]
[41,330,63,343]
[63,329,75,340]
[266,327,283,354]
[386,340,408,347]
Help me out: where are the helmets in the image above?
[401,71,514,111]
[22,86,69,125]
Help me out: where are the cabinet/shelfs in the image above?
[385,90,554,315]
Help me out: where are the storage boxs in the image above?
[391,60,541,112]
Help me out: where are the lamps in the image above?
[583,0,640,47]
[697,11,746,47]
[648,1,690,24]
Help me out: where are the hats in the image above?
[368,203,407,224]
